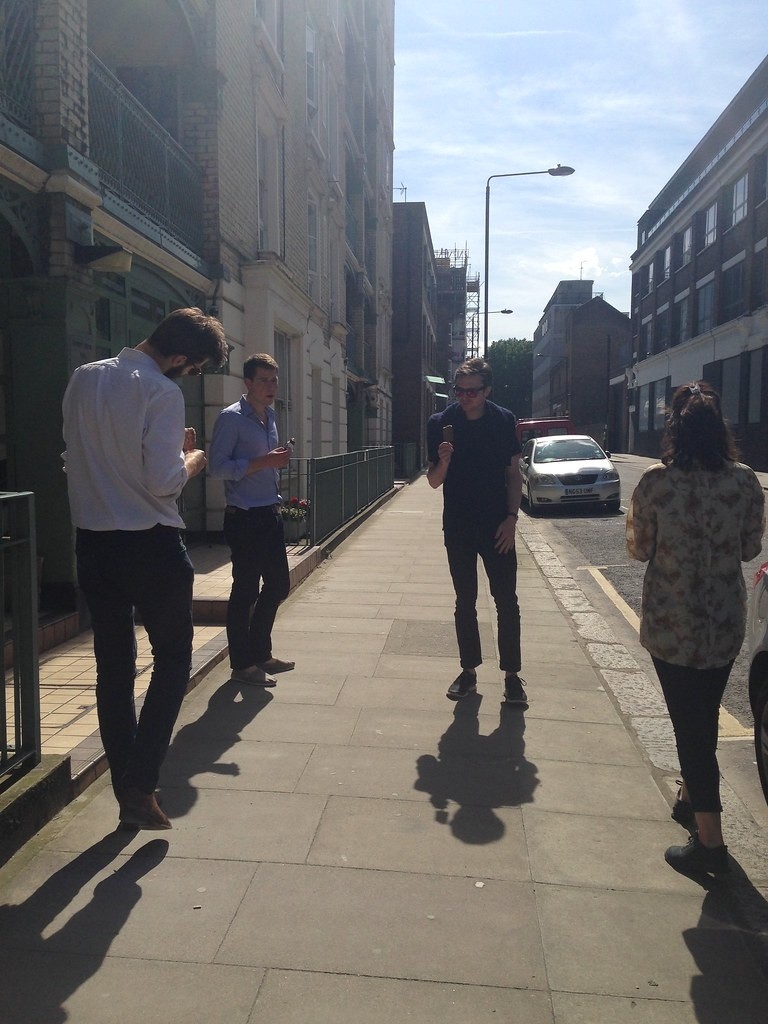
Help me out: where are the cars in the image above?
[519,434,621,515]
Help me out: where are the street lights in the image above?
[484,164,576,363]
[535,353,570,418]
[471,308,513,358]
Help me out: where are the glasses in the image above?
[453,385,485,398]
[188,361,201,377]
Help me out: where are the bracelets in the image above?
[509,511,519,519]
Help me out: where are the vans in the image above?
[515,416,576,451]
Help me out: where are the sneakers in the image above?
[446,671,477,697]
[231,668,277,686]
[119,787,171,830]
[256,659,295,674]
[664,837,729,873]
[504,674,528,703]
[671,787,694,821]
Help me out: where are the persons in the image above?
[207,353,297,688]
[623,383,766,873]
[57,308,232,834]
[424,355,528,703]
